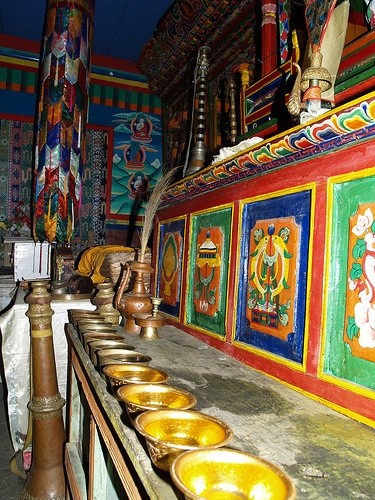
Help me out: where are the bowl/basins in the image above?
[168,448,296,500]
[102,363,169,401]
[96,350,152,381]
[131,312,166,340]
[133,408,234,471]
[116,384,198,424]
[88,340,136,367]
[81,327,118,346]
[82,332,125,355]
[67,309,112,337]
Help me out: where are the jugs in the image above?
[116,261,154,334]
